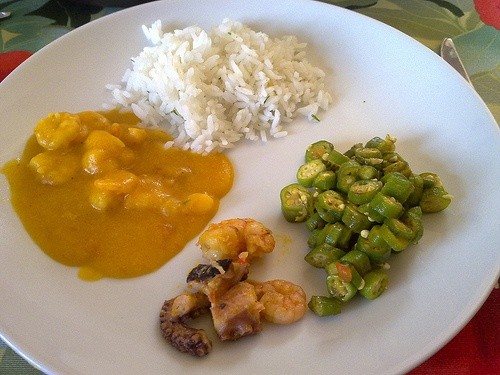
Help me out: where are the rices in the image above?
[104,17,333,157]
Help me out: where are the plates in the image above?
[0,0,500,375]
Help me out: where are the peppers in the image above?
[279,134,453,318]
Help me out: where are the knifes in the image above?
[440,38,473,89]
